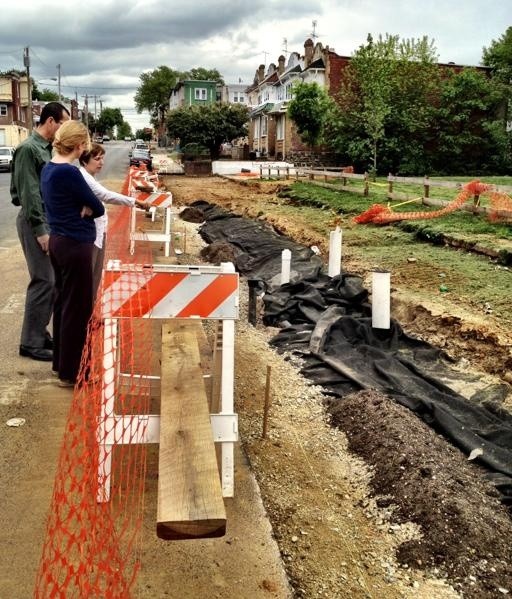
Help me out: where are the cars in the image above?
[93,134,154,169]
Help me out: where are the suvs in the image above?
[0,146,19,172]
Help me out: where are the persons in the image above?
[10,102,71,362]
[40,120,105,388]
[79,144,157,300]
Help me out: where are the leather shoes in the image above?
[19,333,54,361]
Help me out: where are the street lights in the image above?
[28,76,57,135]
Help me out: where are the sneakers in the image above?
[52,369,99,387]
